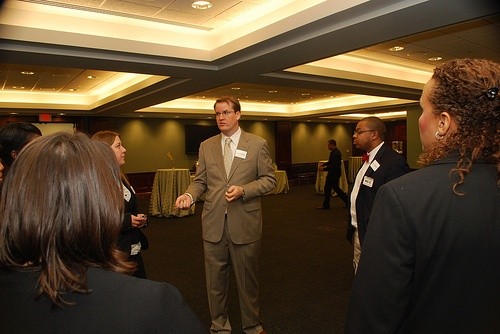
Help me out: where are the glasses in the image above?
[215,110,235,116]
[354,129,381,135]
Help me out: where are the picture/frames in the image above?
[185,124,221,155]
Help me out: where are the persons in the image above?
[316,139,348,210]
[0,122,42,180]
[0,132,214,334]
[344,57,500,334]
[175,96,278,334]
[346,117,412,276]
[91,131,149,275]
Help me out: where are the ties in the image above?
[223,138,234,180]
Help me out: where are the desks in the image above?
[348,157,362,183]
[315,160,348,197]
[263,170,290,195]
[147,168,196,218]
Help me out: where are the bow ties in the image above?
[361,154,369,162]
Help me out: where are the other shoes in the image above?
[316,205,330,209]
